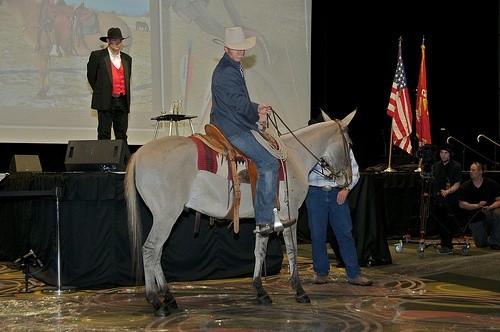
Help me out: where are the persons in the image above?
[423,143,461,254]
[87,28,133,140]
[306,120,373,286]
[209,26,296,235]
[459,162,500,249]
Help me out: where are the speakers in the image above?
[8,154,43,173]
[64,139,132,172]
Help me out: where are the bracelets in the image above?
[344,187,351,192]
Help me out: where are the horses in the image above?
[125,108,357,317]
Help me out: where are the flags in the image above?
[416,49,431,144]
[387,55,414,155]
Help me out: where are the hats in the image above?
[212,26,256,51]
[99,28,130,43]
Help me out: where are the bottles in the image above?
[419,230,426,252]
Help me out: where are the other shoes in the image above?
[348,276,373,285]
[490,244,500,250]
[315,276,328,284]
[259,218,297,236]
